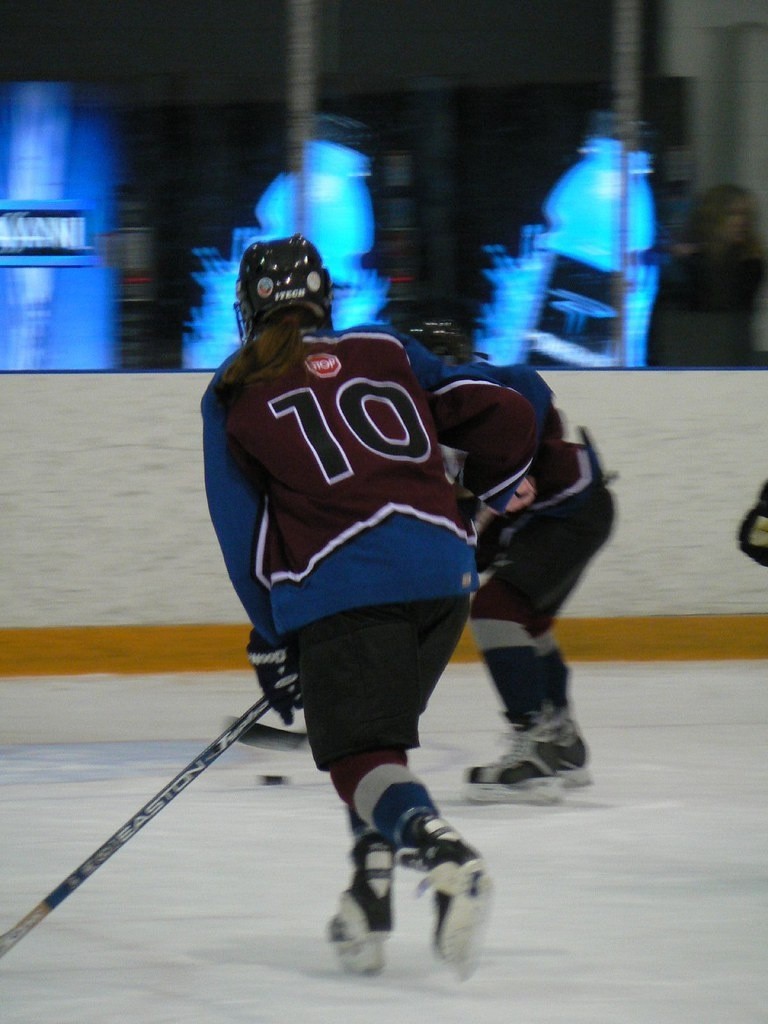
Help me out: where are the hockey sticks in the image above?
[0,695,269,959]
[222,712,308,754]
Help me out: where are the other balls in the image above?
[255,773,286,788]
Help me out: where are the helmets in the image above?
[234,233,333,329]
[399,315,473,367]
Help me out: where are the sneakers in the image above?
[464,709,564,802]
[328,833,393,976]
[395,809,493,984]
[562,705,591,786]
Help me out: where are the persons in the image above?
[200,236,538,977]
[391,301,615,803]
[646,182,768,366]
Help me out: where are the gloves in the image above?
[246,640,302,726]
